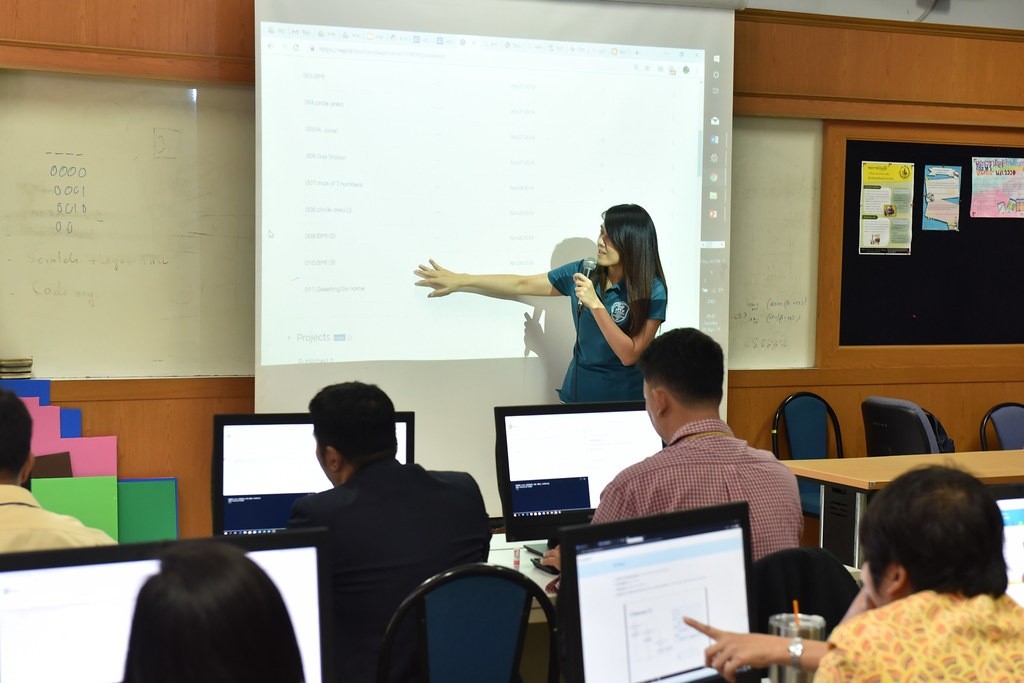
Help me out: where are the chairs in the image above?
[375,563,561,683]
[861,396,955,454]
[772,392,851,517]
[980,400,1024,450]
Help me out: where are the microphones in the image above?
[578,256,597,313]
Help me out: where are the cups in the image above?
[768,613,826,683]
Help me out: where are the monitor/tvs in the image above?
[496,401,668,543]
[986,483,1024,612]
[558,499,765,682]
[211,412,414,535]
[0,528,334,683]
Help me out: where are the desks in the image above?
[775,449,1024,588]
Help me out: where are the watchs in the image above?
[788,634,804,669]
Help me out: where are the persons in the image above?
[811,462,1024,681]
[545,325,806,565]
[413,203,669,406]
[287,381,497,681]
[119,539,308,682]
[1,387,120,553]
[682,560,875,683]
